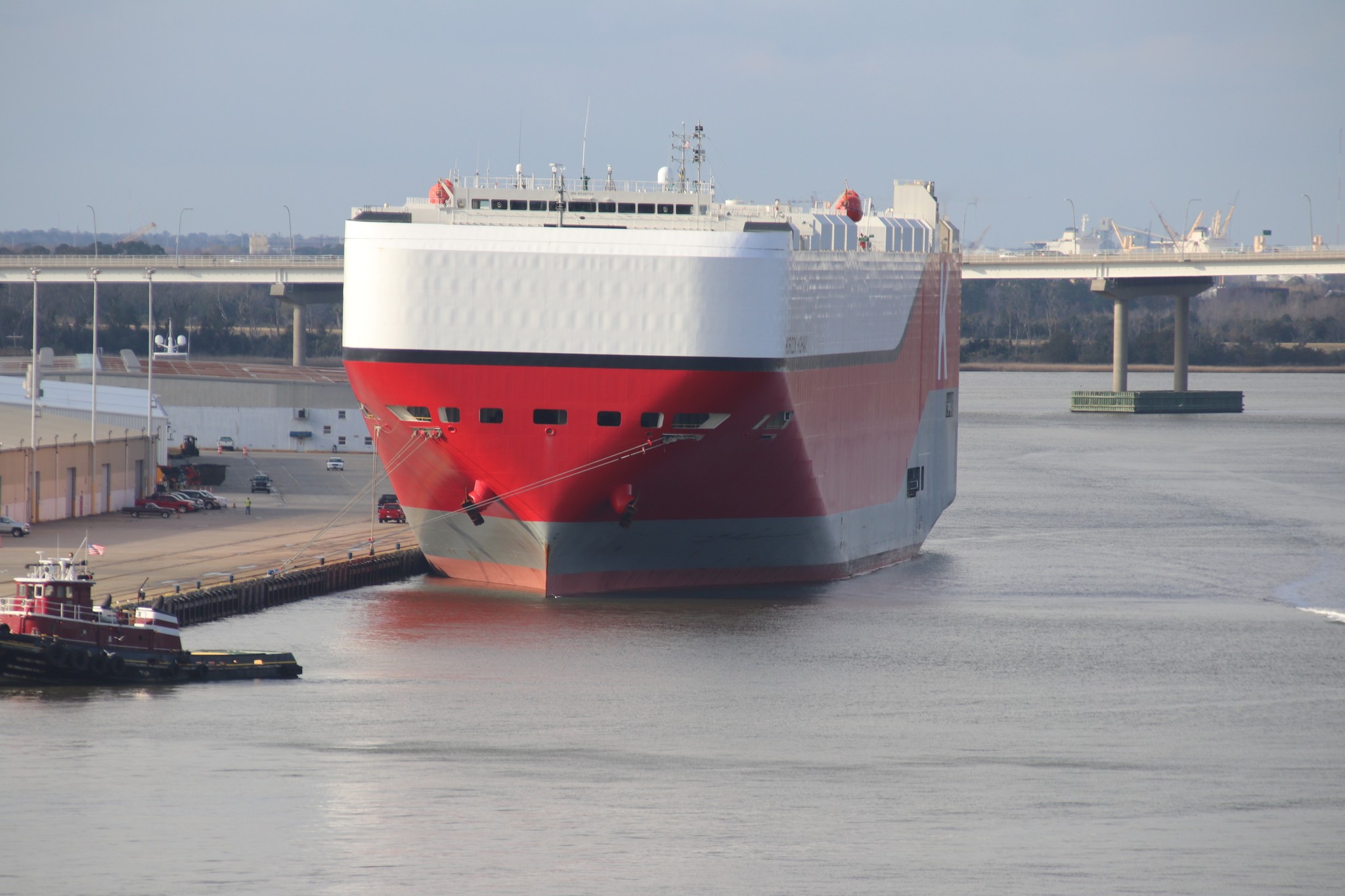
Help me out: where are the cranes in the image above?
[1185,204,1236,241]
[1149,200,1180,252]
[1109,219,1133,249]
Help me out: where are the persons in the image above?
[245,497,251,515]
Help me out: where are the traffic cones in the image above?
[209,485,212,494]
[232,503,237,508]
[199,485,208,490]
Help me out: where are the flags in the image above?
[86,544,105,557]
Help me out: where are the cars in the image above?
[229,258,246,264]
[121,490,227,518]
[326,457,344,471]
[377,494,407,524]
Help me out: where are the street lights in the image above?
[1181,196,1202,263]
[1064,198,1078,258]
[26,265,43,451]
[176,207,194,267]
[144,265,159,440]
[86,268,102,444]
[84,205,99,258]
[281,204,293,263]
[1303,192,1313,247]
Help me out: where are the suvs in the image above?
[249,474,273,493]
[0,514,32,538]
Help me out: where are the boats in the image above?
[340,114,962,595]
[0,536,306,684]
